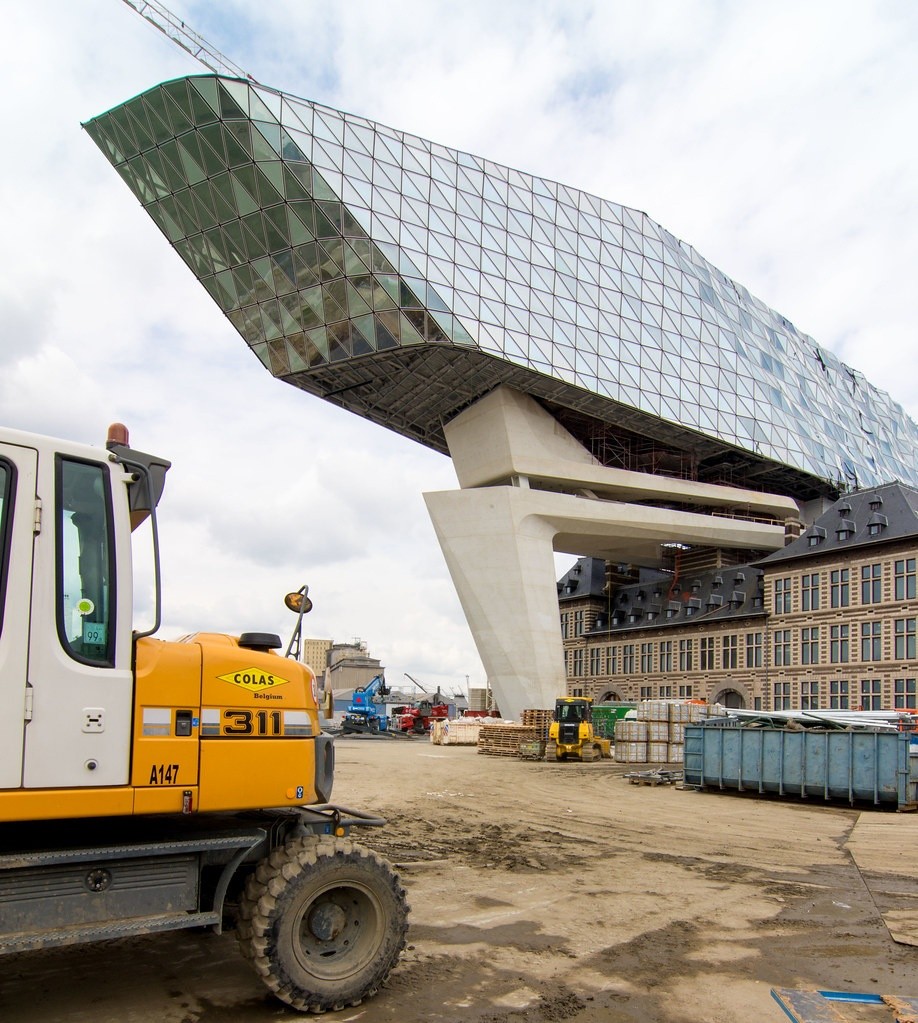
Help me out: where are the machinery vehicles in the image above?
[545,693,611,762]
[340,672,449,739]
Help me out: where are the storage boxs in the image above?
[614,701,724,763]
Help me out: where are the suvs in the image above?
[0,422,412,1017]
